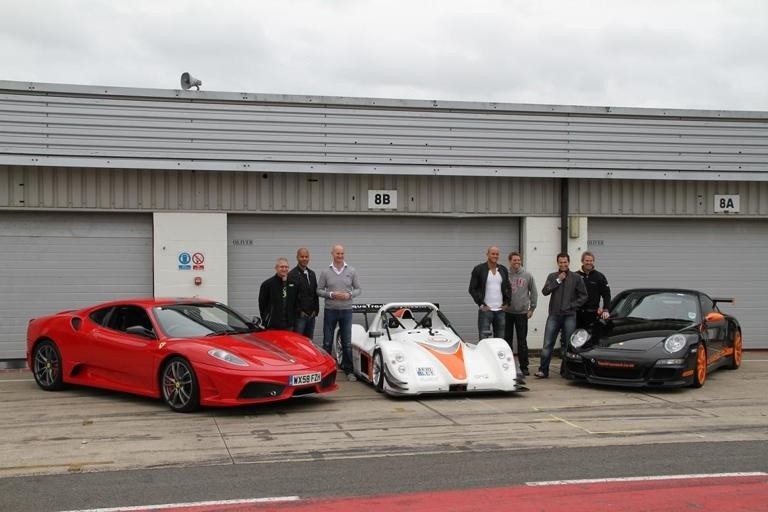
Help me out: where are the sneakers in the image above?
[346,373,357,382]
[533,371,547,378]
[522,369,529,375]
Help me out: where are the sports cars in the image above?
[332,300,522,397]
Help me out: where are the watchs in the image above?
[349,292,351,299]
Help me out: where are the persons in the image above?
[534,253,588,378]
[575,252,611,328]
[504,252,538,375]
[317,245,361,382]
[258,259,290,330]
[469,247,512,341]
[286,248,318,340]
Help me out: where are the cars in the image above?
[26,297,338,412]
[560,287,746,391]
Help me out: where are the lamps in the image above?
[571,216,580,238]
